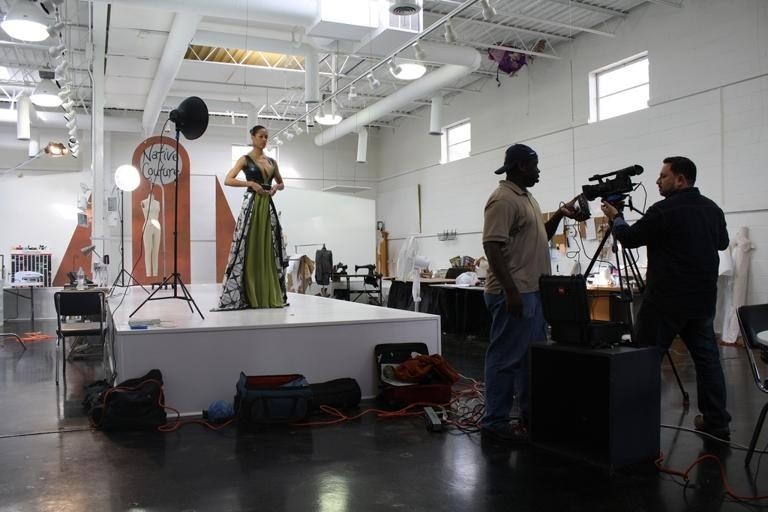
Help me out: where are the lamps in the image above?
[80,246,102,263]
[115,165,140,192]
[169,96,209,140]
[388,47,428,81]
[28,78,62,109]
[314,96,342,126]
[0,0,52,43]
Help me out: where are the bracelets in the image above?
[610,212,624,222]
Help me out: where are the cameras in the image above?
[581,164,646,211]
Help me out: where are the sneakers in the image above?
[694,415,731,439]
[480,423,527,445]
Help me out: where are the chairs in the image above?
[53,289,109,385]
[735,302,768,471]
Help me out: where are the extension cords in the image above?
[424,406,442,425]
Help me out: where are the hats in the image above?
[495,144,538,175]
[456,272,481,287]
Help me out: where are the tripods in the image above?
[566,222,689,401]
[108,192,150,296]
[127,127,206,318]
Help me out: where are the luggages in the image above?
[375,343,451,409]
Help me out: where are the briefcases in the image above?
[538,274,624,349]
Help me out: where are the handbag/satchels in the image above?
[234,372,314,425]
[87,403,106,429]
[81,380,109,409]
[307,378,361,415]
[103,369,180,432]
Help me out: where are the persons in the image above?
[219,126,290,312]
[142,192,161,277]
[601,157,730,435]
[479,143,582,442]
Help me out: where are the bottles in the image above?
[78,267,83,290]
